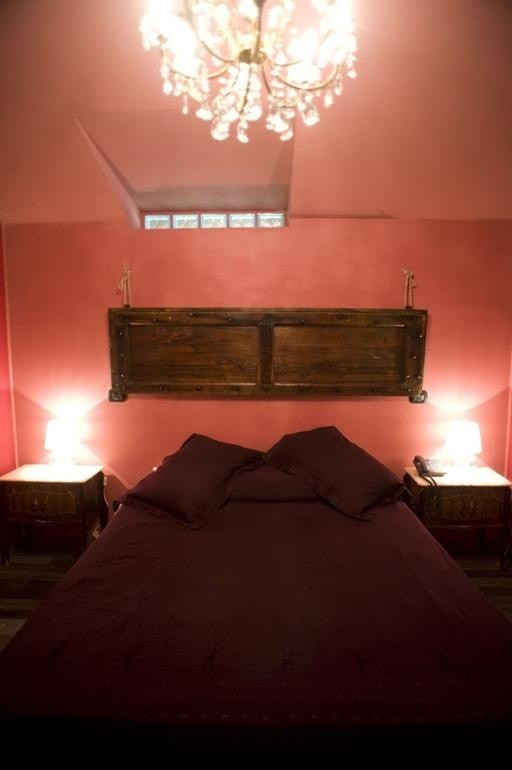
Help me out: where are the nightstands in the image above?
[404,466,511,573]
[0,464,110,565]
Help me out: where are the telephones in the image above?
[413,456,448,476]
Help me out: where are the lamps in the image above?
[140,0,359,142]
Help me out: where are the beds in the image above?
[1,503,510,770]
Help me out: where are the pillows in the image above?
[119,425,409,529]
